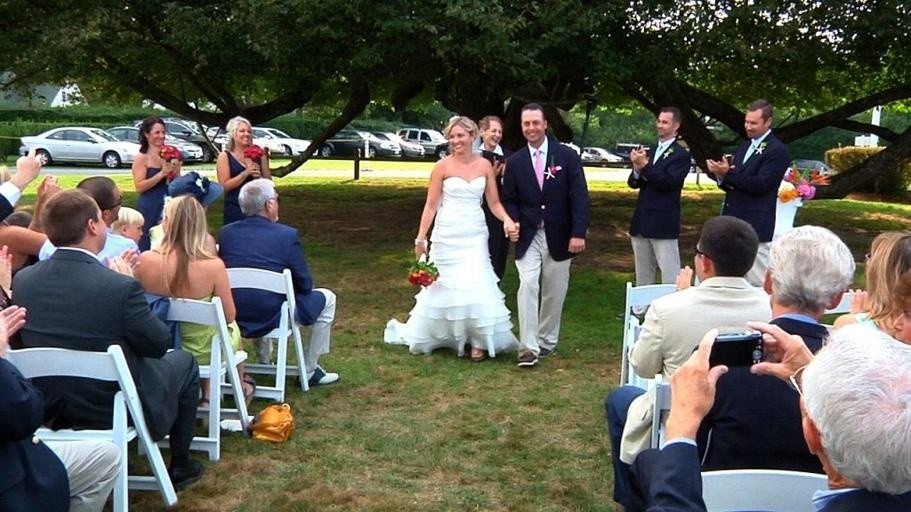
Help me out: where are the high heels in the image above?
[199,397,209,427]
[241,376,255,410]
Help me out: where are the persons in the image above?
[383,116,520,363]
[616,107,691,324]
[503,102,591,367]
[704,100,791,287]
[475,116,516,288]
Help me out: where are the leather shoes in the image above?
[168,459,203,490]
[471,347,487,361]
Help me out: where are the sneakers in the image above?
[517,348,552,367]
[295,365,341,386]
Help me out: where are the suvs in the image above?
[398,128,448,161]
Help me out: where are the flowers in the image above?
[778,156,830,204]
[751,142,767,157]
[662,147,673,160]
[546,155,558,177]
[242,143,262,159]
[406,241,444,290]
[158,143,180,162]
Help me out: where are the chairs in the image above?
[815,290,854,318]
[619,280,685,386]
[5,337,183,512]
[151,292,251,461]
[650,381,676,452]
[695,468,829,512]
[220,263,312,406]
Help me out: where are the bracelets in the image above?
[414,238,427,247]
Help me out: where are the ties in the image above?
[535,150,545,228]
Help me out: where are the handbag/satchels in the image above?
[252,403,294,443]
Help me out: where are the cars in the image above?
[18,126,142,169]
[788,158,837,179]
[136,116,319,167]
[371,131,426,160]
[320,128,402,159]
[102,127,201,163]
[562,142,643,168]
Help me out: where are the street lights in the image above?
[439,120,443,131]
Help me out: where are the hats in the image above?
[167,171,223,207]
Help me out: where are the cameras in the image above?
[709,333,762,369]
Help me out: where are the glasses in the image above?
[789,365,805,400]
